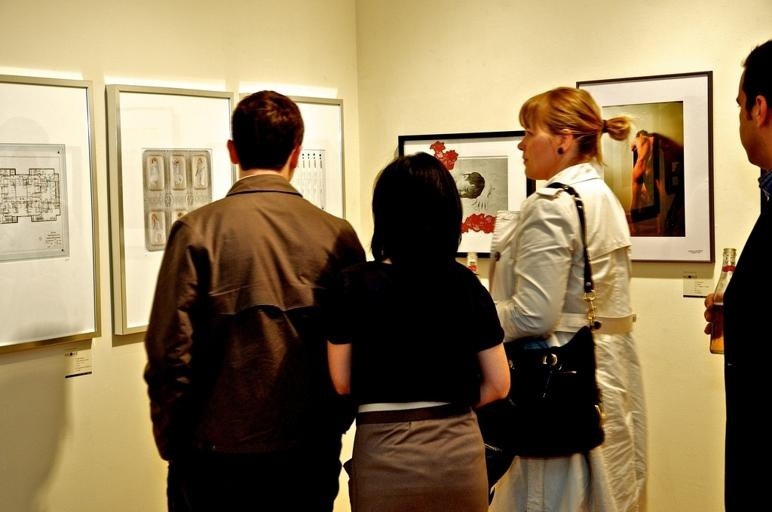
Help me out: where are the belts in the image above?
[355,400,474,424]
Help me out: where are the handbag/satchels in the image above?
[475,315,607,459]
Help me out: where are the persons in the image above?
[488,84,653,511]
[450,171,486,200]
[322,151,516,511]
[630,125,664,234]
[141,88,367,512]
[704,37,772,512]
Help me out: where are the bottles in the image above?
[464,251,480,275]
[706,247,737,356]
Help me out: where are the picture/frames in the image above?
[397,129,536,258]
[573,68,714,264]
